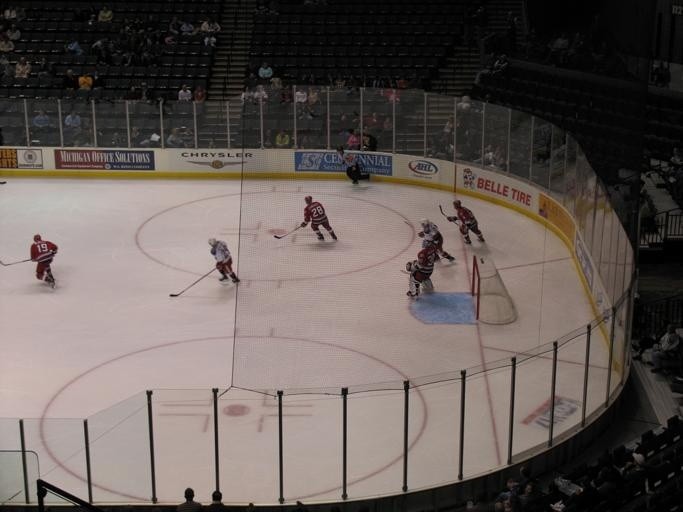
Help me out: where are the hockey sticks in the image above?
[273,226,301,239]
[169,268,216,296]
[0,259,32,266]
[439,205,458,225]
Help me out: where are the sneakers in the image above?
[448,256,455,262]
[316,233,325,241]
[328,231,338,240]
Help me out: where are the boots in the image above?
[463,236,471,244]
[218,272,227,281]
[476,236,485,241]
[229,272,239,283]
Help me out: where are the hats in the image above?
[336,145,343,152]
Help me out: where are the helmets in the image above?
[451,200,461,209]
[208,237,217,247]
[33,234,41,242]
[304,195,312,204]
[420,218,430,227]
[422,240,432,249]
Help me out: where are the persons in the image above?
[630,319,669,361]
[469,451,647,511]
[335,146,369,186]
[447,199,485,244]
[208,237,239,284]
[241,1,444,153]
[174,488,203,510]
[31,233,58,283]
[430,0,683,247]
[407,237,436,298]
[201,490,230,511]
[645,322,680,377]
[419,219,455,263]
[299,195,338,240]
[0,2,221,148]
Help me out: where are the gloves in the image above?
[445,215,456,222]
[300,221,307,228]
